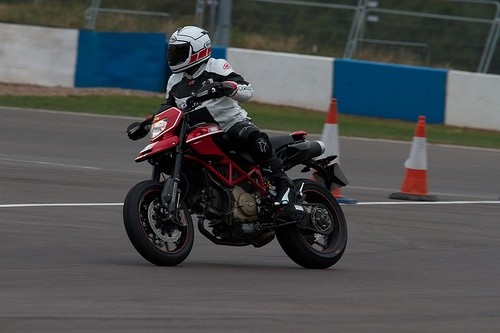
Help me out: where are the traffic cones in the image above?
[388,116,440,202]
[311,97,356,204]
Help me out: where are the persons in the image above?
[127,25,296,207]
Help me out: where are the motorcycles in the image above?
[121,81,348,269]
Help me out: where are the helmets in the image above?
[167,26,212,72]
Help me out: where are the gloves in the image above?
[126,122,149,140]
[197,82,234,103]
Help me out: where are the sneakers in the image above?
[272,185,295,207]
